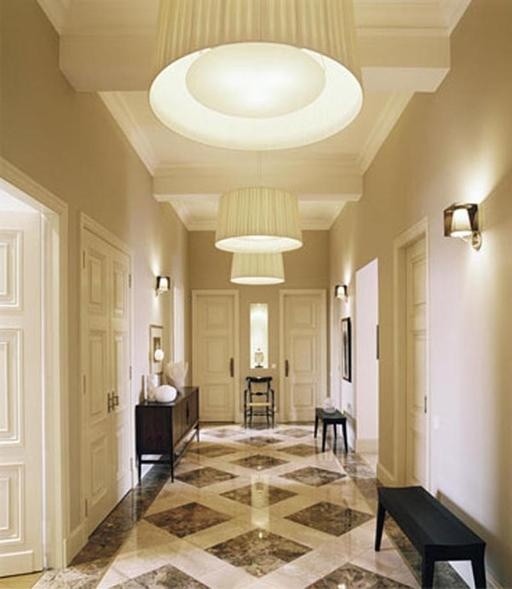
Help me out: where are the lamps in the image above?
[213,152,303,253]
[442,202,482,250]
[335,285,349,305]
[230,251,286,286]
[155,275,171,296]
[148,0,365,151]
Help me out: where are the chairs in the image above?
[244,376,275,428]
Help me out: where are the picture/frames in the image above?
[341,317,351,383]
[149,325,164,375]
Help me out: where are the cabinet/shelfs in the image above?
[135,385,200,483]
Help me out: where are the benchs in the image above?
[375,485,487,589]
[314,407,348,452]
